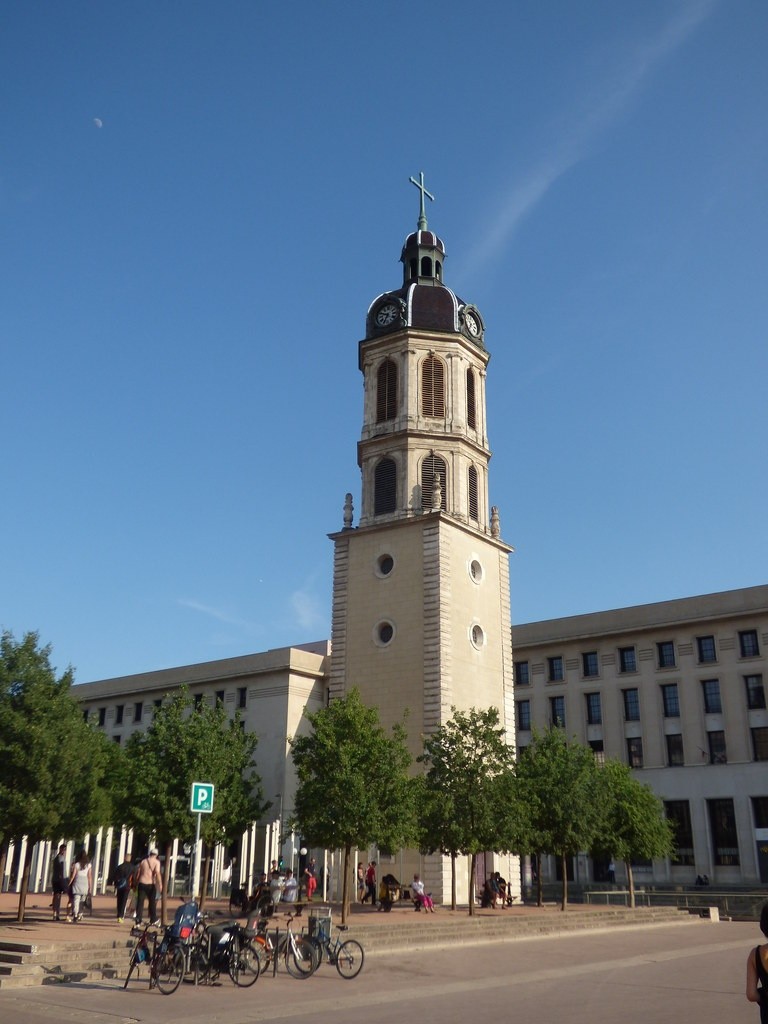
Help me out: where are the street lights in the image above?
[275,792,283,870]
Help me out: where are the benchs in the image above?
[475,895,517,906]
[265,901,312,917]
[384,884,433,911]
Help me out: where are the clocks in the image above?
[377,303,399,325]
[465,314,478,336]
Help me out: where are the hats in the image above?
[150,849,158,855]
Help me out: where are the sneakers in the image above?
[117,917,124,924]
[152,919,160,925]
[136,922,144,927]
[72,917,78,923]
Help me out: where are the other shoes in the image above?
[53,916,57,920]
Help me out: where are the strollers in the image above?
[61,877,93,917]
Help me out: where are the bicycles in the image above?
[120,900,364,996]
[228,874,276,919]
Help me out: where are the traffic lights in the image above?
[278,855,283,867]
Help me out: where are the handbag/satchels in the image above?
[85,894,92,909]
[116,878,128,890]
[230,887,241,905]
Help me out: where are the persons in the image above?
[252,855,331,917]
[67,848,92,922]
[607,861,615,884]
[695,875,709,886]
[353,861,378,905]
[409,872,437,914]
[109,854,134,923]
[480,872,510,909]
[746,900,768,1024]
[51,843,66,921]
[136,848,162,927]
[378,874,401,912]
